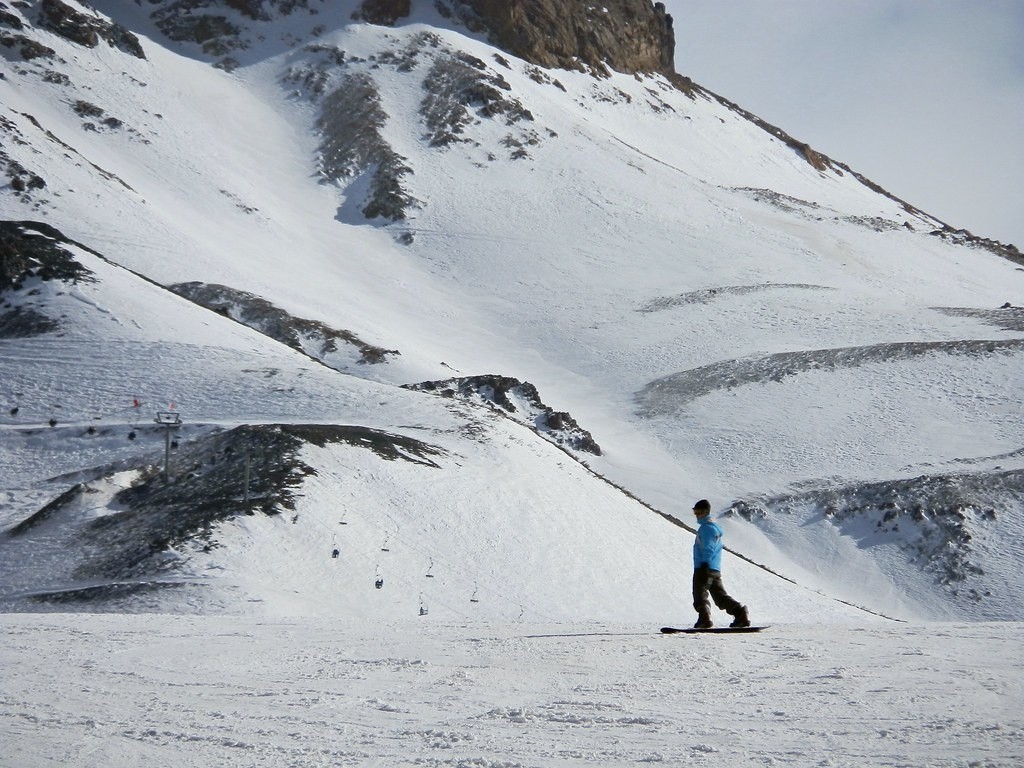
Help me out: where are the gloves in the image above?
[700,562,708,573]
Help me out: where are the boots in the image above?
[729,605,750,627]
[694,608,713,628]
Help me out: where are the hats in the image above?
[692,500,711,512]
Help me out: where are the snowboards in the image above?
[662,625,770,635]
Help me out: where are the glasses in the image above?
[693,510,702,515]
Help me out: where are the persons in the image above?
[692,499,751,627]
